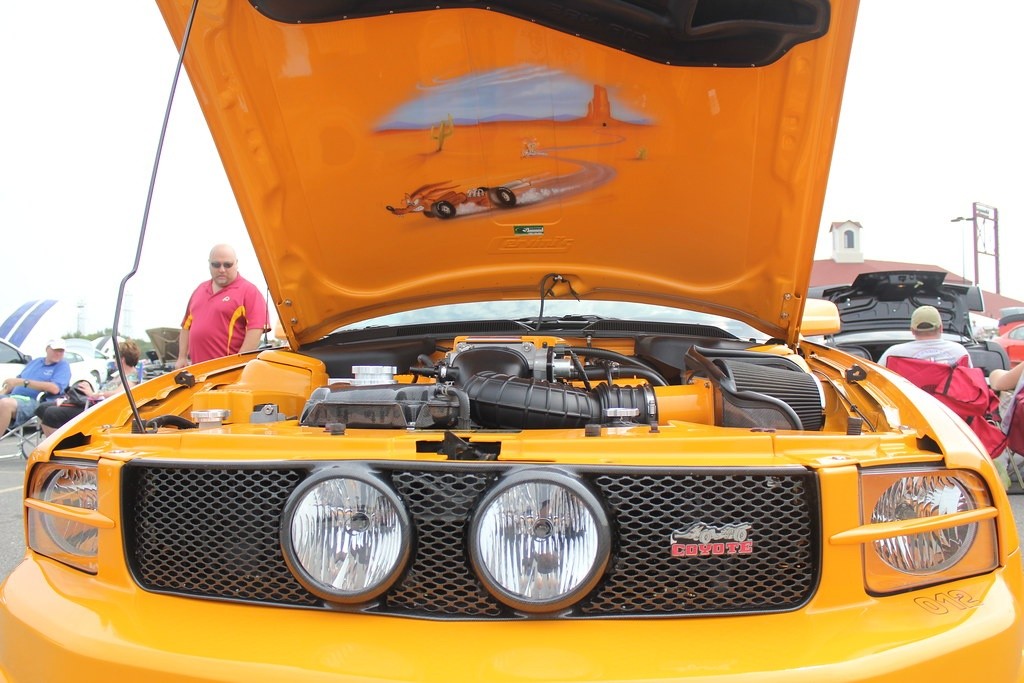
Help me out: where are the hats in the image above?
[45,338,66,350]
[911,306,942,332]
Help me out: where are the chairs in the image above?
[884,353,1024,496]
[0,362,143,460]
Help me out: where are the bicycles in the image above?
[0,299,181,396]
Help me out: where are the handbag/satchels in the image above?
[66,380,95,405]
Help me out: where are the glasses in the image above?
[210,263,235,269]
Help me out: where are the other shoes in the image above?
[1005,481,1024,494]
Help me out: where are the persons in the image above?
[989,362,1024,390]
[877,305,973,368]
[275,321,288,347]
[0,339,72,436]
[175,244,272,369]
[143,356,149,364]
[35,341,140,438]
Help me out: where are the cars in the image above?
[0,0,1024,683]
[807,270,1024,377]
